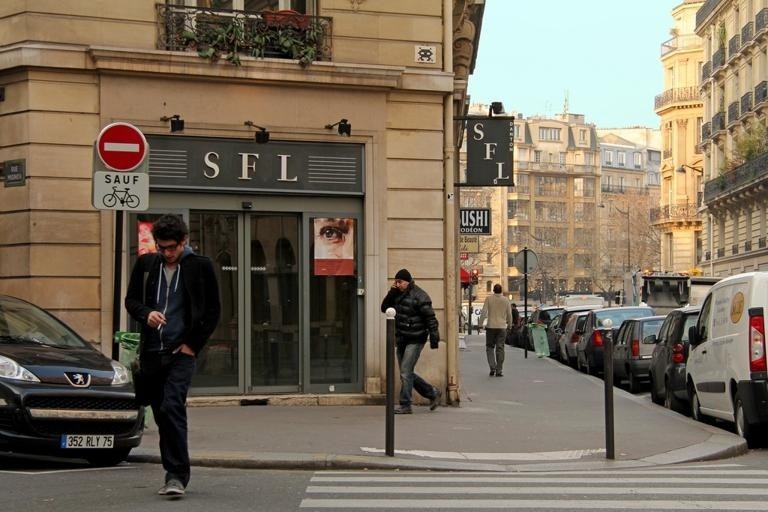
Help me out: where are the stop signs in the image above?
[98,122,146,171]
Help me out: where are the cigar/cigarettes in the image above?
[156,321,162,331]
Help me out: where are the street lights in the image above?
[676,162,714,278]
[514,228,544,277]
[595,200,631,272]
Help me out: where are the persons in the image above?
[510,302,522,344]
[379,266,442,412]
[316,218,354,260]
[124,211,220,498]
[478,283,513,380]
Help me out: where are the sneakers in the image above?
[158,480,185,495]
[489,368,496,376]
[394,406,412,414]
[430,390,442,411]
[495,374,503,377]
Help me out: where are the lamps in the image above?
[244,120,272,144]
[486,100,506,119]
[321,118,353,137]
[160,113,188,135]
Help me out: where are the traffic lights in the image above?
[471,268,477,284]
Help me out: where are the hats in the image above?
[394,269,412,283]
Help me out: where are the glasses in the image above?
[155,242,180,251]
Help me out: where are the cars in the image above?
[1,294,147,467]
[189,314,353,382]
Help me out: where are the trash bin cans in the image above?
[114,331,154,428]
[524,323,551,358]
